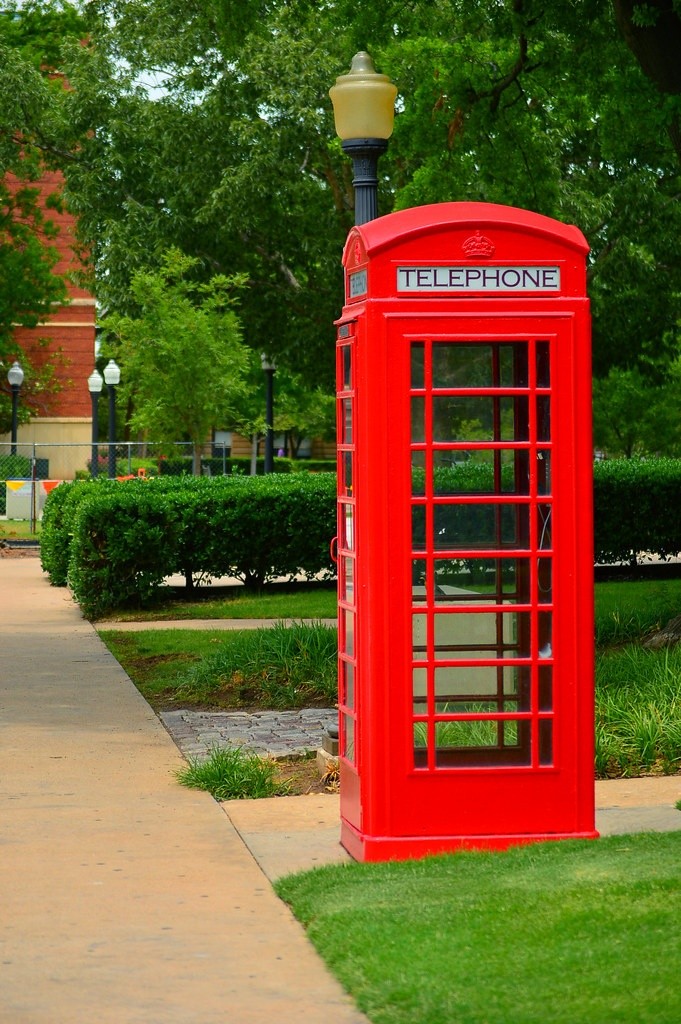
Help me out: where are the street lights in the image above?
[326,51,398,227]
[260,341,276,474]
[7,360,25,456]
[104,357,119,479]
[87,367,105,477]
